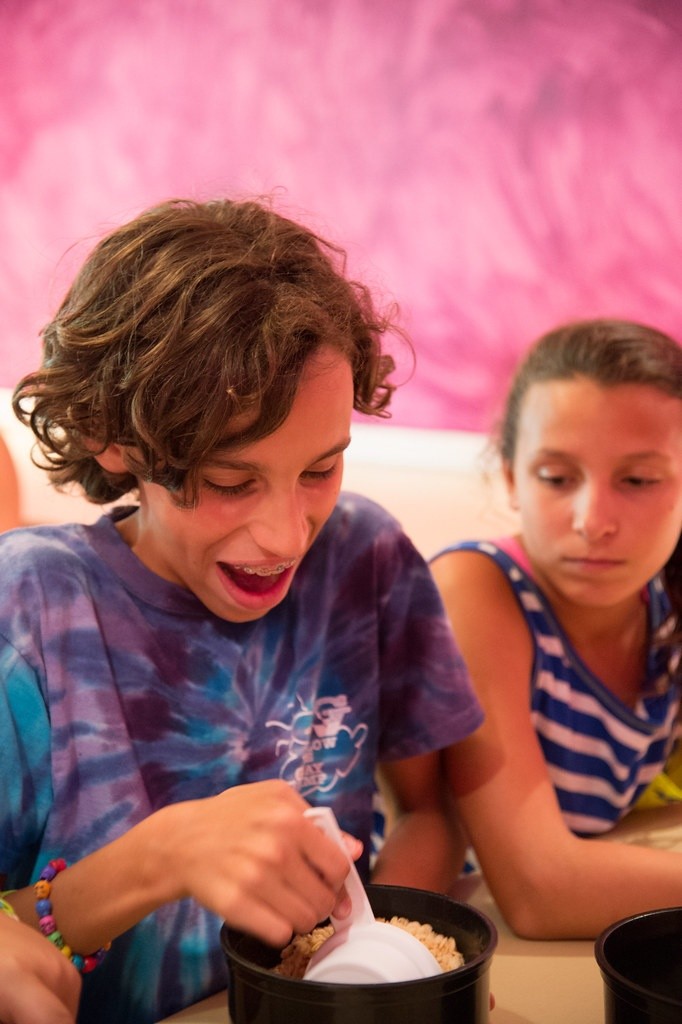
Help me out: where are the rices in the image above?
[270,915,464,975]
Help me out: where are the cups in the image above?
[219,883,499,1024]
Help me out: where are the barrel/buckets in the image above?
[594,905,681,1023]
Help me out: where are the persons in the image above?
[0,197,487,1024]
[365,317,682,941]
[0,897,82,1024]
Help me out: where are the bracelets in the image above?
[0,889,19,921]
[34,858,111,973]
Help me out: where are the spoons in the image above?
[301,806,443,983]
